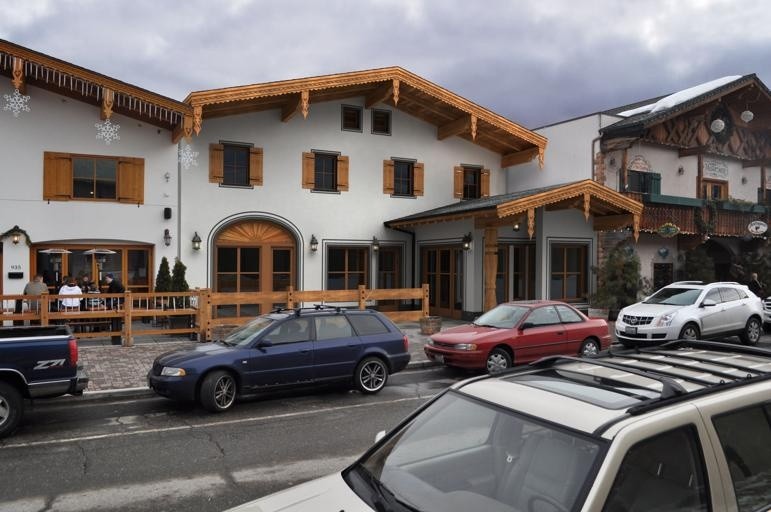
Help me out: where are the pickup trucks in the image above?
[1,326,89,440]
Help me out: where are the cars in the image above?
[615,279,764,346]
[423,299,612,374]
[147,304,410,413]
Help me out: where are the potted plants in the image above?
[579,236,642,321]
[152,255,191,337]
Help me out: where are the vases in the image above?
[419,315,441,335]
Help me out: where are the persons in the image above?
[23,274,127,332]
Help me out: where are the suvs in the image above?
[222,340,771,511]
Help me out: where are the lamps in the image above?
[372,236,379,252]
[309,234,317,251]
[163,229,171,247]
[512,224,520,232]
[191,232,201,250]
[8,225,20,245]
[461,232,472,250]
